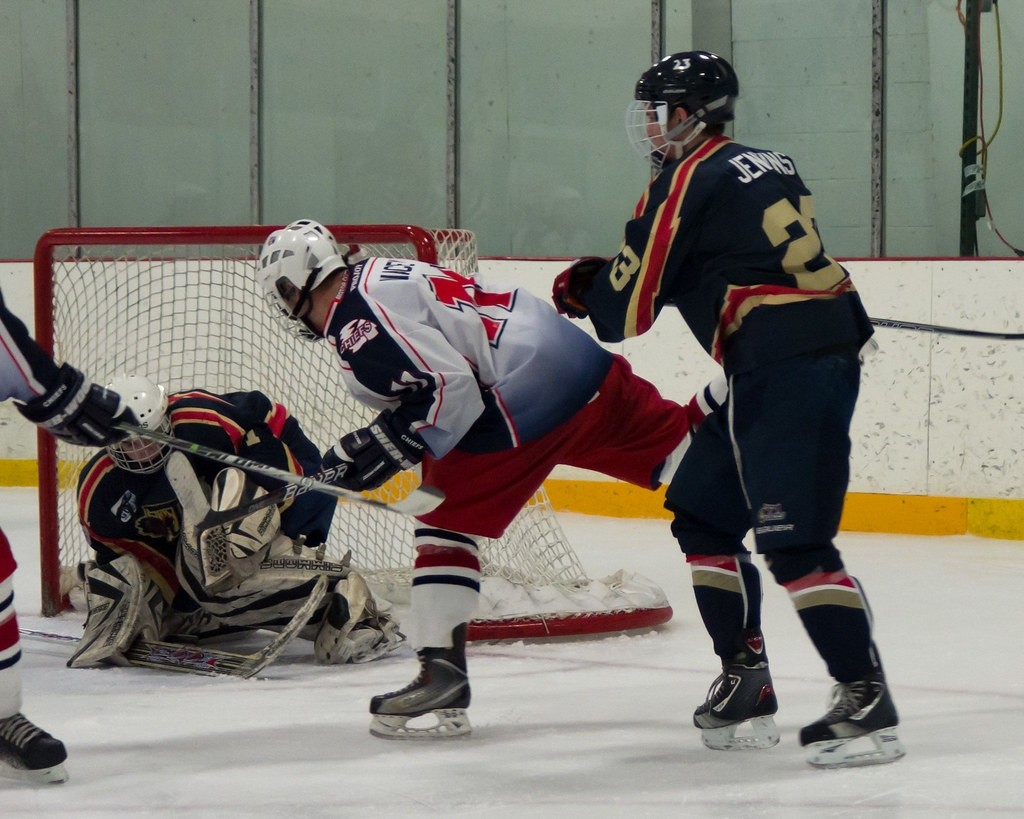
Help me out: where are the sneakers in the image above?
[0,714,70,781]
[313,573,405,671]
[797,674,905,766]
[695,661,783,751]
[368,621,473,740]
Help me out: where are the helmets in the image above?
[259,219,350,293]
[102,373,170,442]
[637,50,738,138]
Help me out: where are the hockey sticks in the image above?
[7,575,328,681]
[195,458,349,588]
[868,316,1024,342]
[109,420,447,516]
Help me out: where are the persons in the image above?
[262,222,730,739]
[80,376,394,657]
[551,54,906,768]
[0,290,136,785]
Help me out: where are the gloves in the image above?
[550,256,606,320]
[14,361,137,447]
[316,409,425,493]
